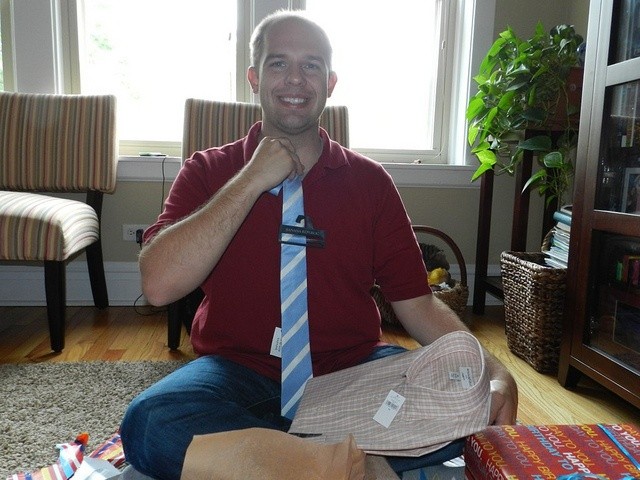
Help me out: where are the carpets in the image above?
[0,360,196,480]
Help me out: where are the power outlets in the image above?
[122,224,149,242]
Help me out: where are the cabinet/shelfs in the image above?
[559,1,639,408]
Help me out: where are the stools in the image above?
[463,423,640,480]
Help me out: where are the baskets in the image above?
[373,225,468,325]
[501,251,568,373]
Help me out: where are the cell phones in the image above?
[140,152,165,156]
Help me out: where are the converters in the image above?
[136,229,143,243]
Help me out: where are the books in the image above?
[542,206,571,270]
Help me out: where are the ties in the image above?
[270,178,314,420]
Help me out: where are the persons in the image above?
[118,8,519,480]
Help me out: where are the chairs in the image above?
[166,96,261,350]
[318,105,351,150]
[0,91,117,350]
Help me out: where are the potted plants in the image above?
[465,21,586,213]
